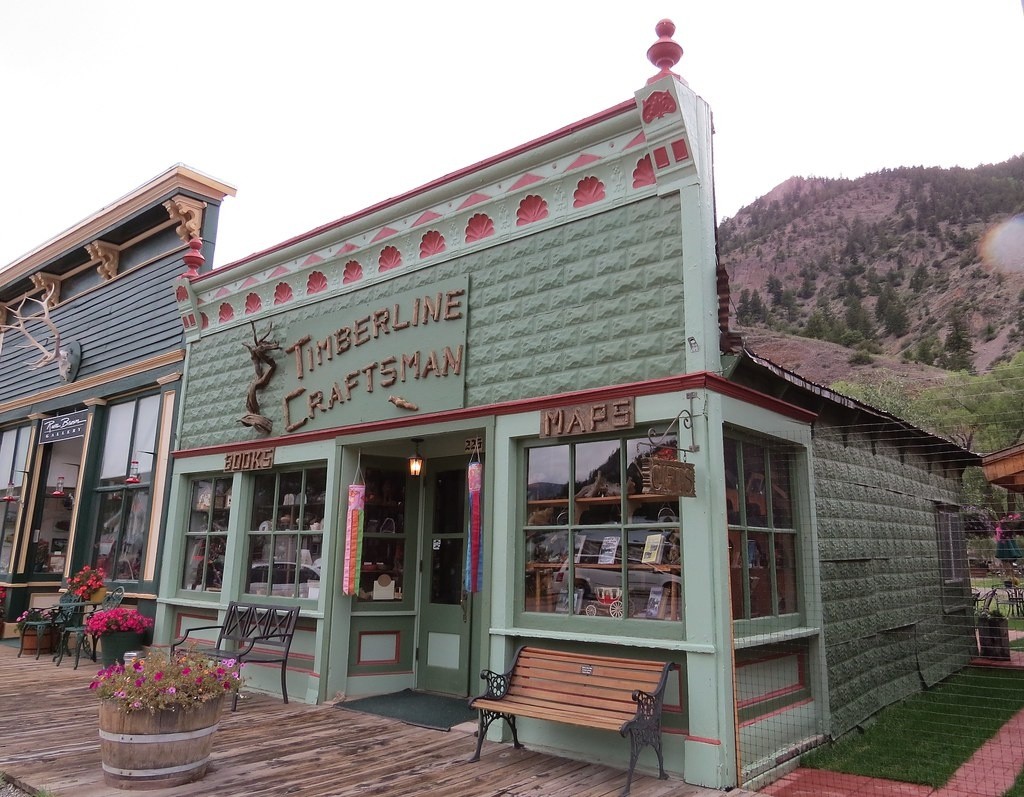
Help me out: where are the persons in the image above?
[106,499,146,558]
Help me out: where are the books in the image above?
[646,587,671,620]
[560,535,587,564]
[640,534,666,563]
[555,589,584,615]
[598,536,621,565]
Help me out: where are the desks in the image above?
[997,586,1024,619]
[51,601,102,663]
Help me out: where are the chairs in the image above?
[17,591,83,660]
[56,586,126,669]
[972,584,1024,618]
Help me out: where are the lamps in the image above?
[50,462,80,496]
[408,437,426,475]
[125,450,155,483]
[2,470,29,502]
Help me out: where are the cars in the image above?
[248,563,328,599]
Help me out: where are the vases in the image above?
[101,699,221,790]
[100,629,150,667]
[90,586,108,601]
[0,618,4,634]
[20,620,62,656]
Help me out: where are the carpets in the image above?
[332,688,478,733]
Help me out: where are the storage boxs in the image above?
[299,580,320,600]
[250,583,268,595]
[272,584,294,597]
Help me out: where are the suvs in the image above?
[556,553,683,601]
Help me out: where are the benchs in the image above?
[467,646,680,797]
[173,601,301,711]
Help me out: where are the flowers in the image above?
[66,566,108,600]
[90,645,248,714]
[14,607,56,632]
[0,587,7,616]
[85,607,152,637]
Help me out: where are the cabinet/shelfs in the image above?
[525,495,682,620]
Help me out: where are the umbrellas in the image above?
[996,521,1023,586]
